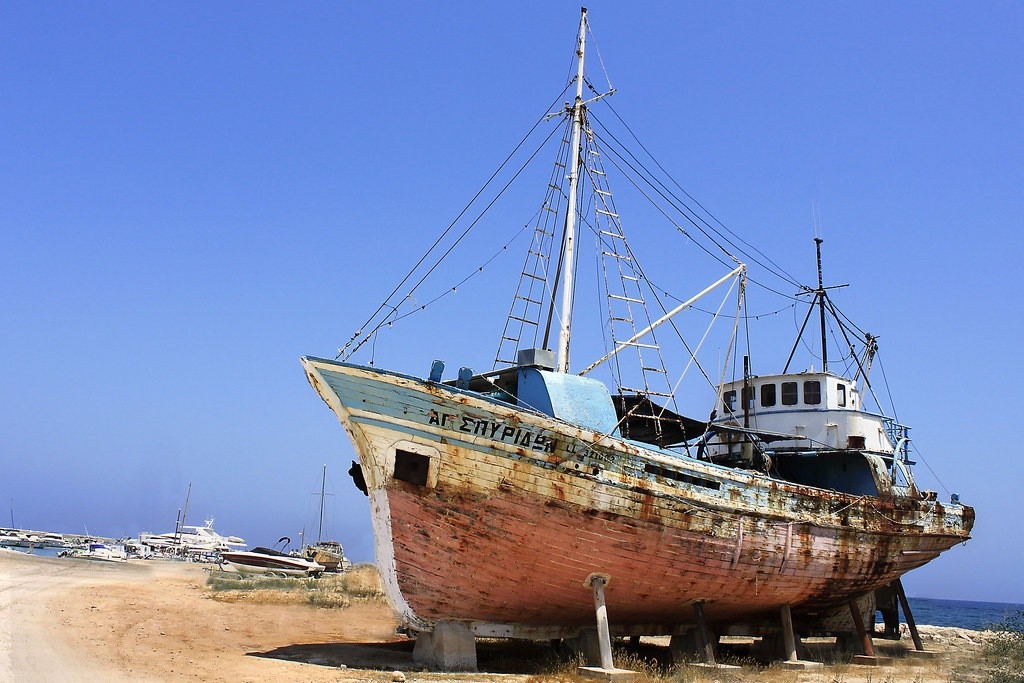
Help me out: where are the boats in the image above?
[0,463,351,580]
[298,4,978,642]
